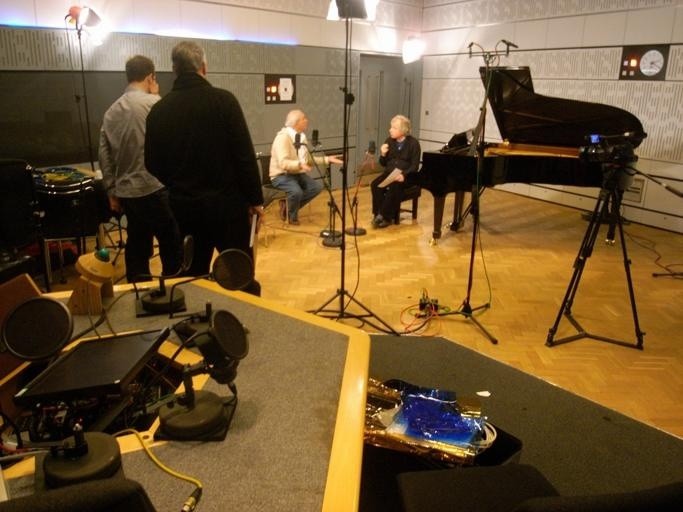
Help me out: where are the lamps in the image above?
[310,0,399,337]
[65,5,117,170]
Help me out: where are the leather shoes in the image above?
[279,199,299,224]
[372,215,392,227]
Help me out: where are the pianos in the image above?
[405,65,650,247]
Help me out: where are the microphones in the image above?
[312,130,318,147]
[167,248,255,354]
[131,309,250,442]
[0,296,74,361]
[368,141,376,155]
[295,134,300,149]
[132,234,194,317]
[468,42,473,58]
[502,40,519,48]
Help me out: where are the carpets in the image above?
[365,333,683,512]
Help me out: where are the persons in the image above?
[144,40,266,298]
[269,108,345,227]
[369,113,420,228]
[98,54,185,287]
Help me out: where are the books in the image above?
[250,212,257,249]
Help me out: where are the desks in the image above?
[0,276,371,512]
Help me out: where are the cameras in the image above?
[579,131,648,162]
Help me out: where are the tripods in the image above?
[415,51,499,345]
[314,3,401,337]
[544,162,683,351]
[104,216,126,265]
[77,28,127,251]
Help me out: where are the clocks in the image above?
[617,43,670,81]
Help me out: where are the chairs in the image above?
[253,150,300,224]
[385,162,424,224]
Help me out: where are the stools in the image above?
[39,234,89,293]
